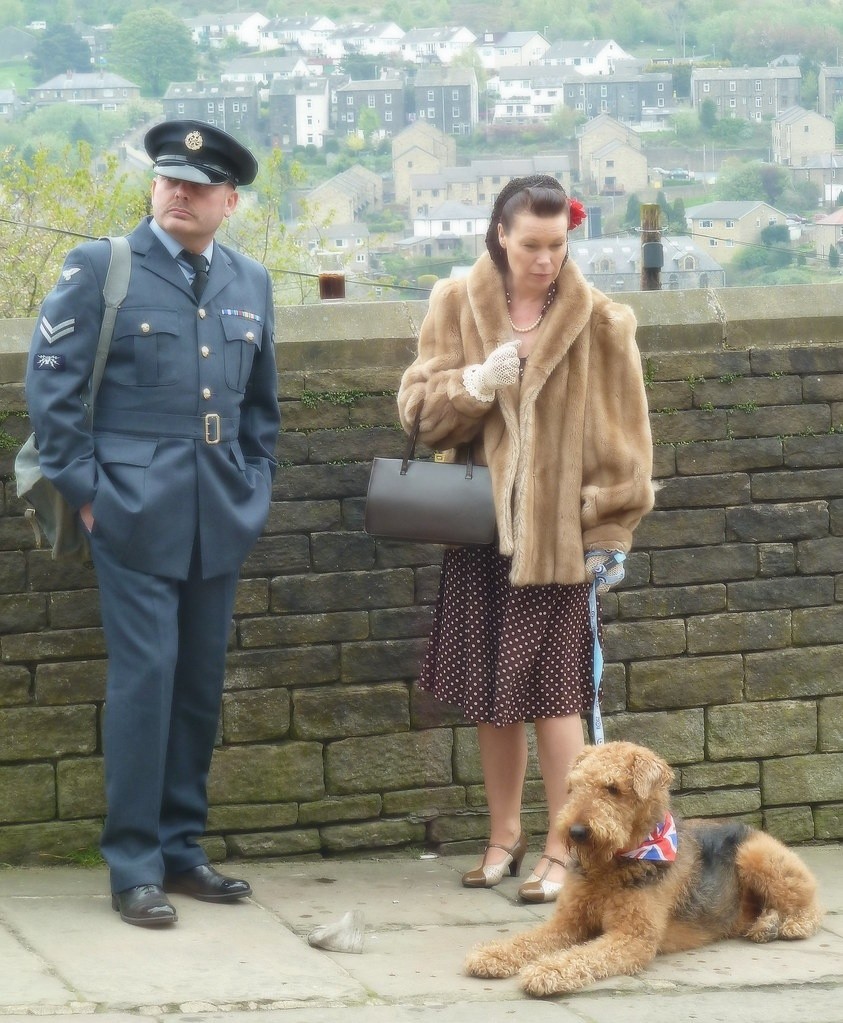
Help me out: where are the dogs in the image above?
[465,740,819,994]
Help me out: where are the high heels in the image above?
[519,854,573,901]
[462,832,527,887]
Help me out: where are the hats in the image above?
[144,120,259,186]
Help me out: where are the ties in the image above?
[181,251,209,303]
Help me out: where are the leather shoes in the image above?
[112,884,178,926]
[163,864,253,902]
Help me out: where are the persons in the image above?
[396,175,656,906]
[25,120,279,924]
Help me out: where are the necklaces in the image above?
[504,280,555,333]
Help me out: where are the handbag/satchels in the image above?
[15,236,131,563]
[365,400,496,545]
